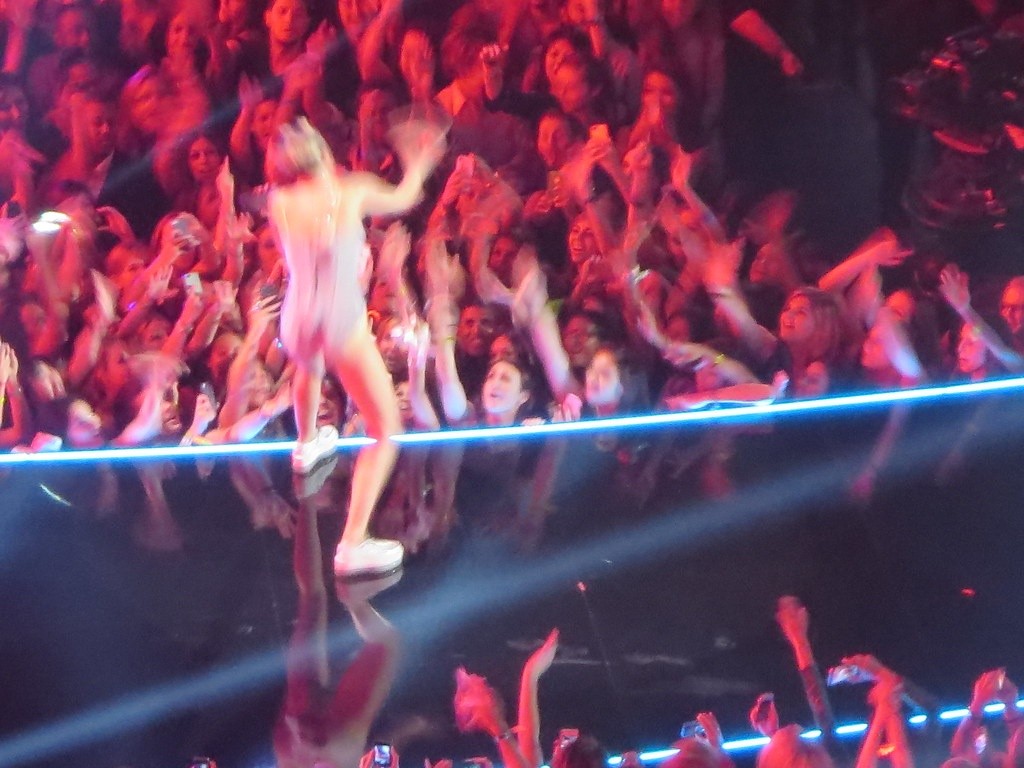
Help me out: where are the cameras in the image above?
[171,220,200,247]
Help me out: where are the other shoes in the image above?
[334,530,403,576]
[291,422,339,474]
[292,454,338,498]
[334,566,406,606]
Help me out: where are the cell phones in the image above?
[6,202,21,219]
[261,284,282,323]
[372,740,393,768]
[201,384,215,412]
[561,393,583,420]
[193,757,210,768]
[559,729,580,754]
[181,272,204,294]
[682,722,705,739]
[590,124,611,143]
[827,664,858,686]
[752,694,774,727]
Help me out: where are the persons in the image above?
[0,0,1024,453]
[270,455,397,768]
[262,117,447,578]
[186,595,1024,768]
[85,390,1014,555]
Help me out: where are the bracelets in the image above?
[177,320,193,333]
[966,713,983,721]
[1003,716,1020,724]
[144,290,157,303]
[584,193,599,206]
[629,196,651,208]
[710,353,725,368]
[974,321,987,334]
[8,385,23,397]
[496,731,512,744]
[228,251,244,257]
